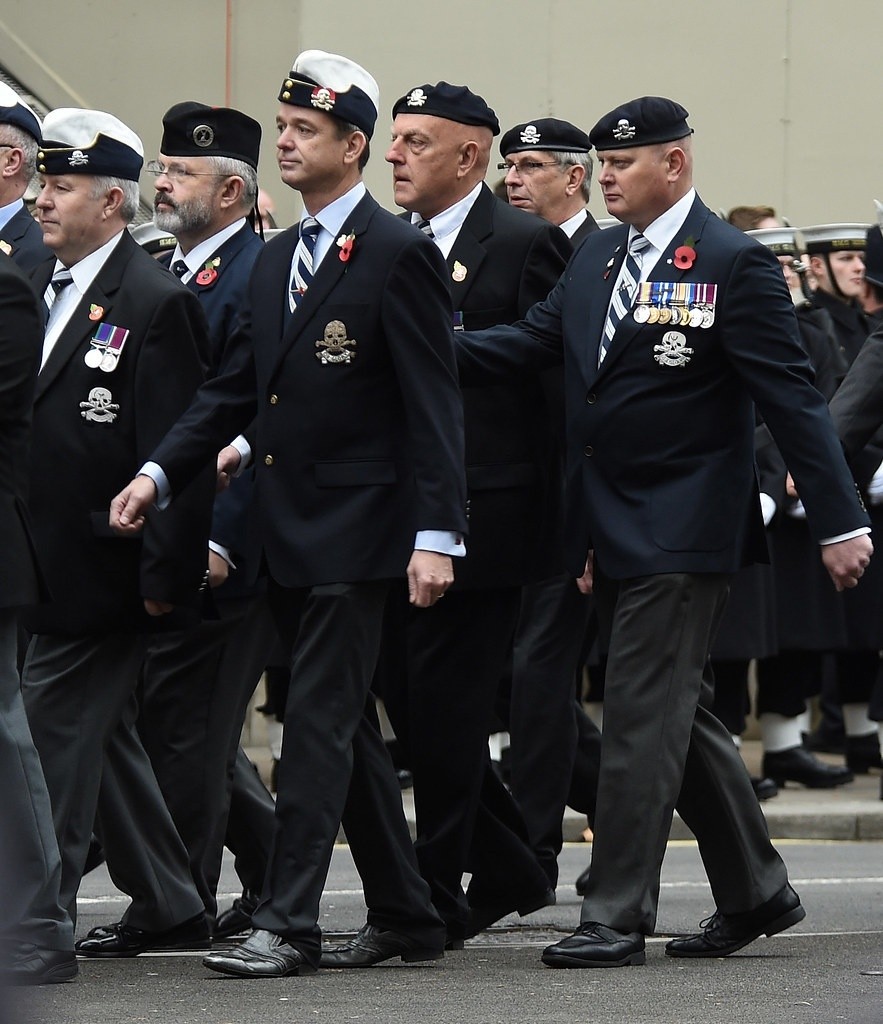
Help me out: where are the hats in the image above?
[0,82,42,144]
[500,118,592,157]
[589,96,696,150]
[392,79,501,137]
[35,104,144,183]
[160,101,263,173]
[744,198,883,289]
[277,49,380,139]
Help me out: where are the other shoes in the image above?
[747,725,882,800]
[466,876,555,938]
[574,863,592,896]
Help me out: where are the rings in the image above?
[436,593,444,597]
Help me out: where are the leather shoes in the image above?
[666,879,806,958]
[86,920,122,938]
[321,916,445,964]
[3,941,80,984]
[215,888,258,940]
[202,926,304,977]
[540,920,646,966]
[77,911,212,959]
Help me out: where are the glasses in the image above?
[498,162,570,175]
[145,160,243,182]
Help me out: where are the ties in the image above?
[43,266,74,332]
[416,221,435,241]
[173,261,188,281]
[289,217,322,315]
[597,235,650,372]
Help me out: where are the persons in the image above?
[0,48,883,990]
